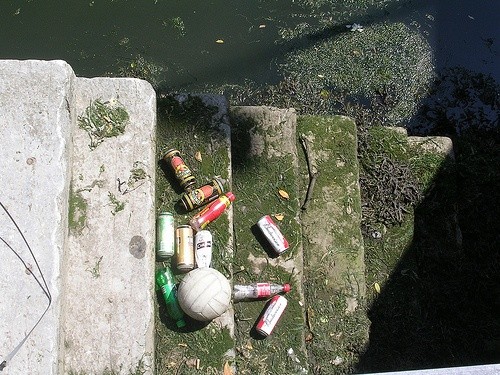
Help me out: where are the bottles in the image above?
[195,230,212,269]
[189,192,235,231]
[232,281,291,300]
[156,267,186,328]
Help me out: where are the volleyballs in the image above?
[178,267,232,322]
